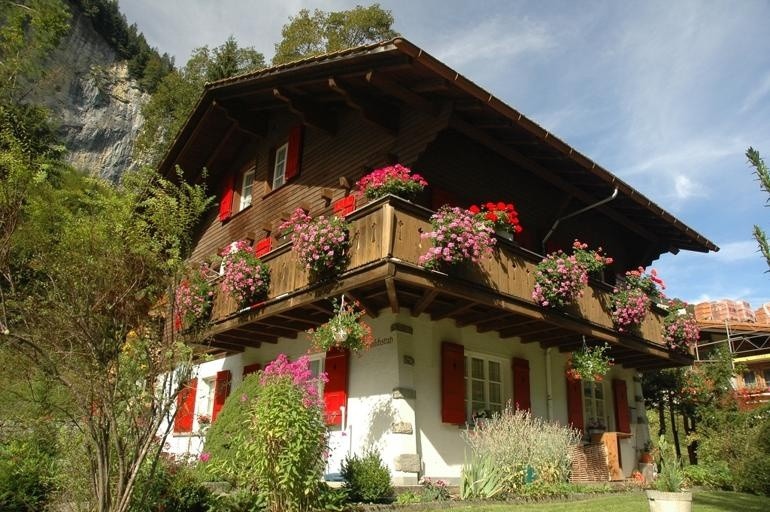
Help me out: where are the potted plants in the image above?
[196,414,210,433]
[645,464,694,512]
[641,440,656,463]
[589,421,606,443]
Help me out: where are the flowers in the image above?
[174,164,700,386]
